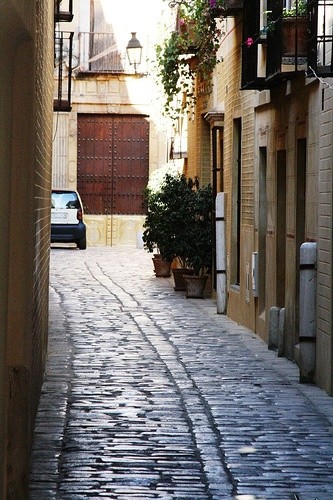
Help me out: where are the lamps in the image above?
[126,31,143,75]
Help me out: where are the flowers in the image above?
[156,0,229,119]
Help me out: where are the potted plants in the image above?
[142,172,216,299]
[263,0,308,57]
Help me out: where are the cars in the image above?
[50,189,86,250]
